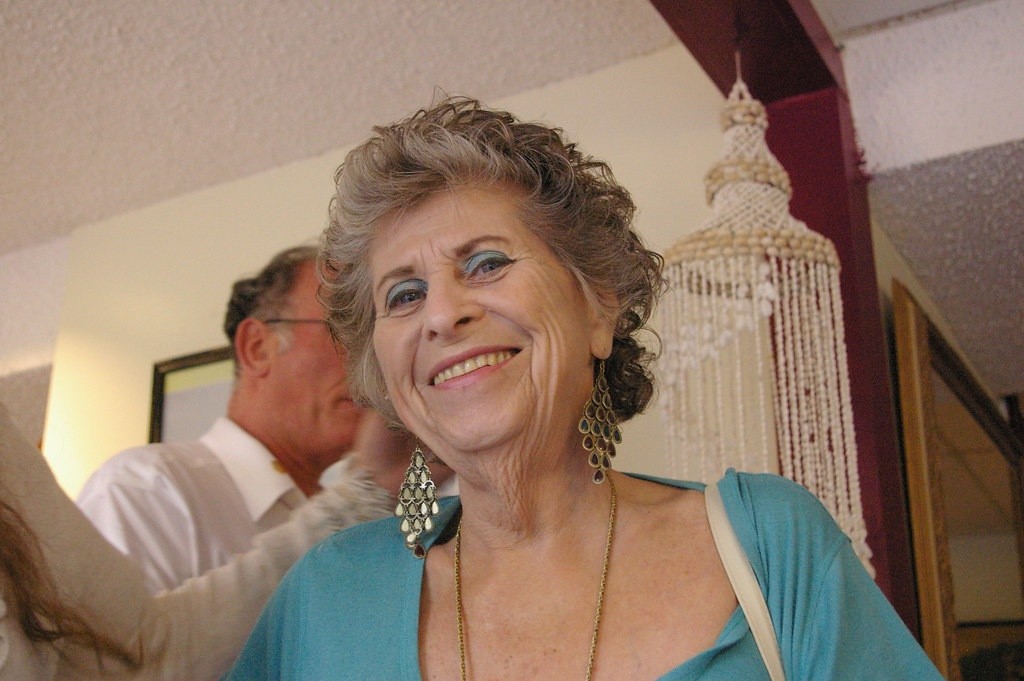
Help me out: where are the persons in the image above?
[0,401,451,681]
[221,85,946,681]
[73,244,368,598]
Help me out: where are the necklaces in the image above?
[454,473,616,680]
